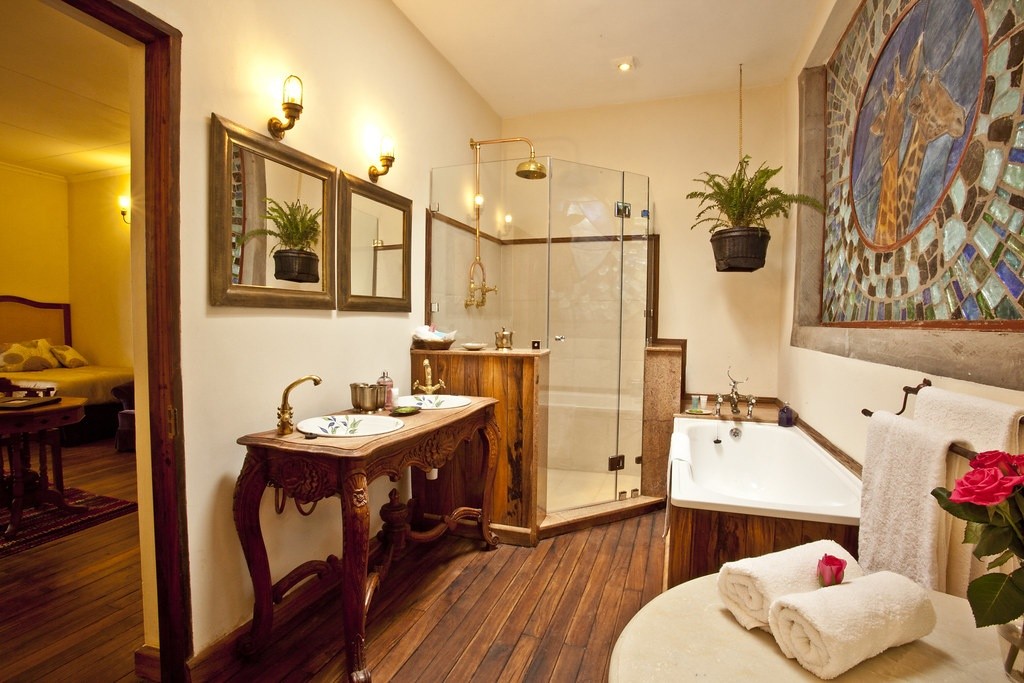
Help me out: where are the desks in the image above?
[0,395,90,537]
[233,392,499,683]
[605,568,1024,683]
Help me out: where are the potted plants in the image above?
[234,193,322,283]
[688,152,825,276]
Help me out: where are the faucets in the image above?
[412,359,447,395]
[728,383,740,413]
[276,374,323,435]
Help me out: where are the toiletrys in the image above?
[689,395,709,413]
[777,401,795,427]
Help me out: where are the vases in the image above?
[995,613,1024,683]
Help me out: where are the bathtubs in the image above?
[667,417,866,591]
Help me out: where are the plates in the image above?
[391,406,420,413]
[685,409,713,414]
[462,343,488,349]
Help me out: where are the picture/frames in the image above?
[821,0,1024,333]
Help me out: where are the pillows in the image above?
[0,340,42,371]
[51,344,89,367]
[34,337,62,368]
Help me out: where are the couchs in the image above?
[109,381,137,452]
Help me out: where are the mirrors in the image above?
[205,113,337,312]
[340,166,416,313]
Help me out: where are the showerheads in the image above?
[515,160,547,180]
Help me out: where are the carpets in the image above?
[0,470,139,558]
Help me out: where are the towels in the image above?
[715,538,937,681]
[661,431,694,538]
[856,385,1024,597]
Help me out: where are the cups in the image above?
[371,384,387,411]
[350,383,378,414]
[495,327,514,350]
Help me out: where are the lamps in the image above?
[367,135,396,185]
[115,194,130,225]
[267,74,306,142]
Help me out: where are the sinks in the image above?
[295,414,406,437]
[393,394,472,409]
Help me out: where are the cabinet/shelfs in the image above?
[410,345,553,548]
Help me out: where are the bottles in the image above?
[378,372,393,411]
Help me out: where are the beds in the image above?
[0,295,135,446]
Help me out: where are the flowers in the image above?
[816,554,847,587]
[930,446,1024,627]
